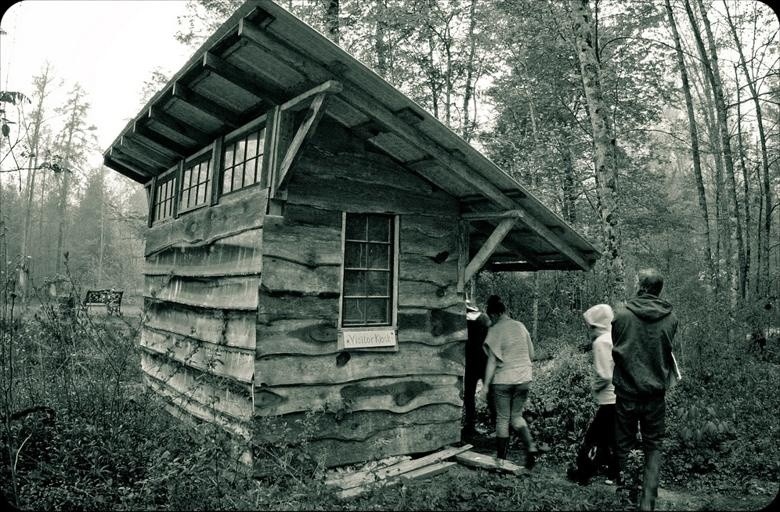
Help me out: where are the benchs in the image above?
[83,289,125,317]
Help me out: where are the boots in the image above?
[518,426,538,470]
[495,436,509,461]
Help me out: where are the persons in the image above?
[483,295,535,469]
[572,302,615,486]
[465,299,495,444]
[611,267,679,511]
